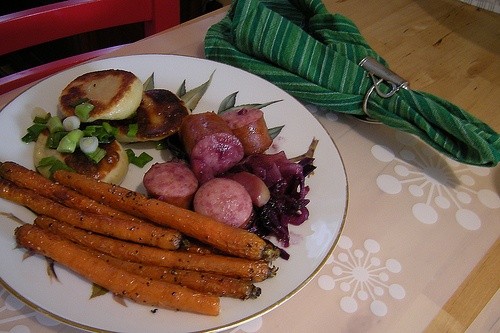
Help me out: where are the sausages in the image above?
[144,106,275,231]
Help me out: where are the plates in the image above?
[0,53,349,333]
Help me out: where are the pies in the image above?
[59,69,143,122]
[34,127,130,188]
[107,89,189,142]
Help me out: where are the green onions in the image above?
[22,102,154,175]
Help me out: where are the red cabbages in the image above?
[228,137,319,261]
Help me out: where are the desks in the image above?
[0,0,500,333]
